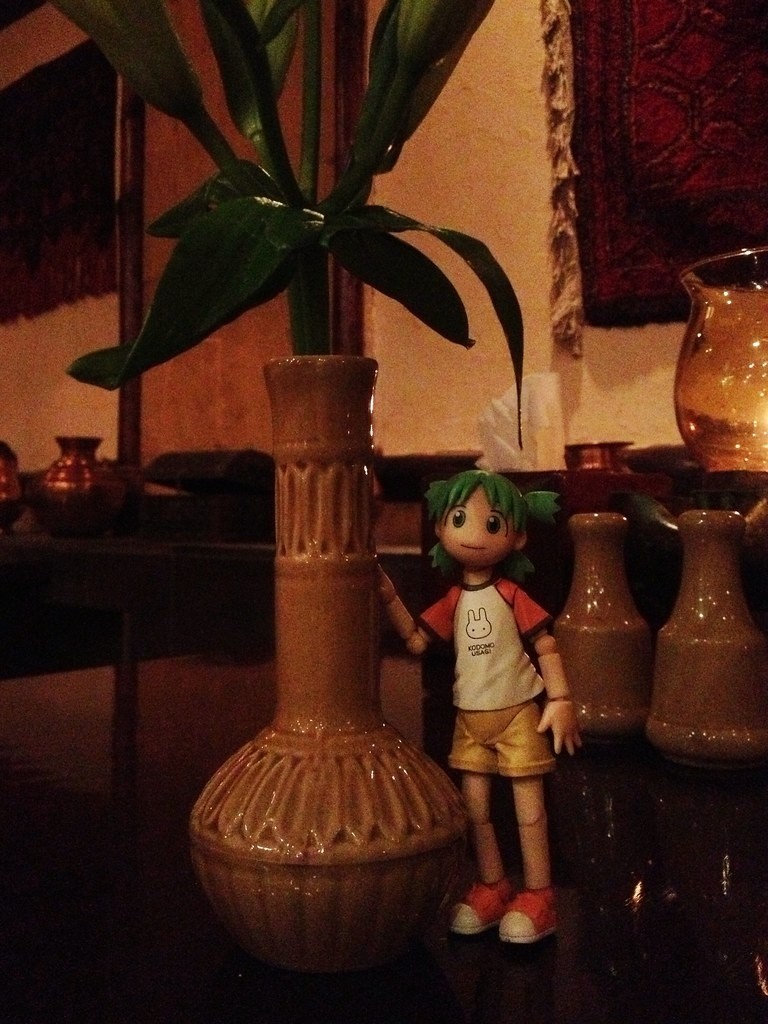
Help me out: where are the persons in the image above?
[374,468,582,941]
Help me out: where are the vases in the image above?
[190,352,464,975]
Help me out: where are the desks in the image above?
[0,532,421,659]
[0,644,768,1024]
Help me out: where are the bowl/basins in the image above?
[369,451,485,502]
[564,440,634,472]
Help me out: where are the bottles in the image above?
[38,435,126,540]
[673,247,768,474]
